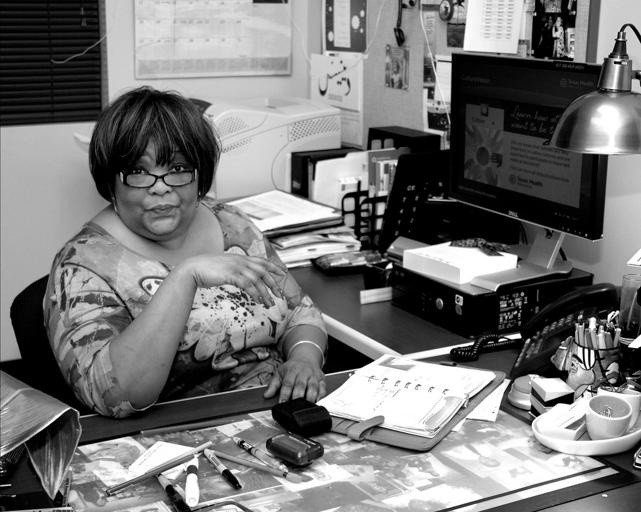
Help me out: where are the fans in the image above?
[134,1,294,80]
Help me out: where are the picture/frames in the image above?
[290,264,521,363]
[64,348,641,512]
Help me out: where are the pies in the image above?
[213,451,303,483]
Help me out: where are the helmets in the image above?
[533,403,641,454]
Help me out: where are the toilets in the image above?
[448,283,619,379]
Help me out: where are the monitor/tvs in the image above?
[220,189,362,270]
[403,242,519,287]
[315,353,507,453]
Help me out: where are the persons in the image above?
[323,444,441,496]
[42,85,329,420]
[389,61,403,89]
[434,418,501,468]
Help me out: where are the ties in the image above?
[445,49,605,289]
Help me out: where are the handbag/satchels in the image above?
[286,340,326,369]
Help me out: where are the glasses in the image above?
[546,24,641,156]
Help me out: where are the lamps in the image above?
[8,273,90,415]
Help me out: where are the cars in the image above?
[586,396,632,439]
[599,388,640,428]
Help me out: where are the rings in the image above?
[204,448,242,489]
[158,473,191,511]
[107,454,195,496]
[146,441,213,474]
[141,419,236,436]
[233,436,289,473]
[576,310,622,349]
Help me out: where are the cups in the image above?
[119,169,197,188]
